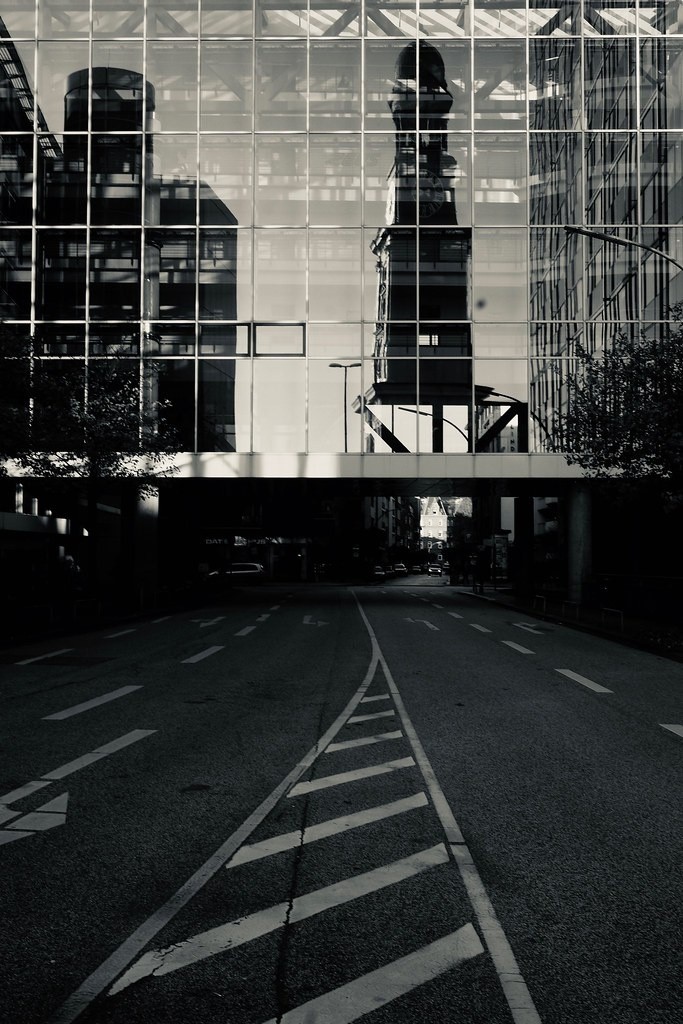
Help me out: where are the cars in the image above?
[373,563,422,579]
[428,563,443,577]
[208,562,264,576]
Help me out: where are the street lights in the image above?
[491,389,556,451]
[328,361,361,453]
[398,406,468,443]
